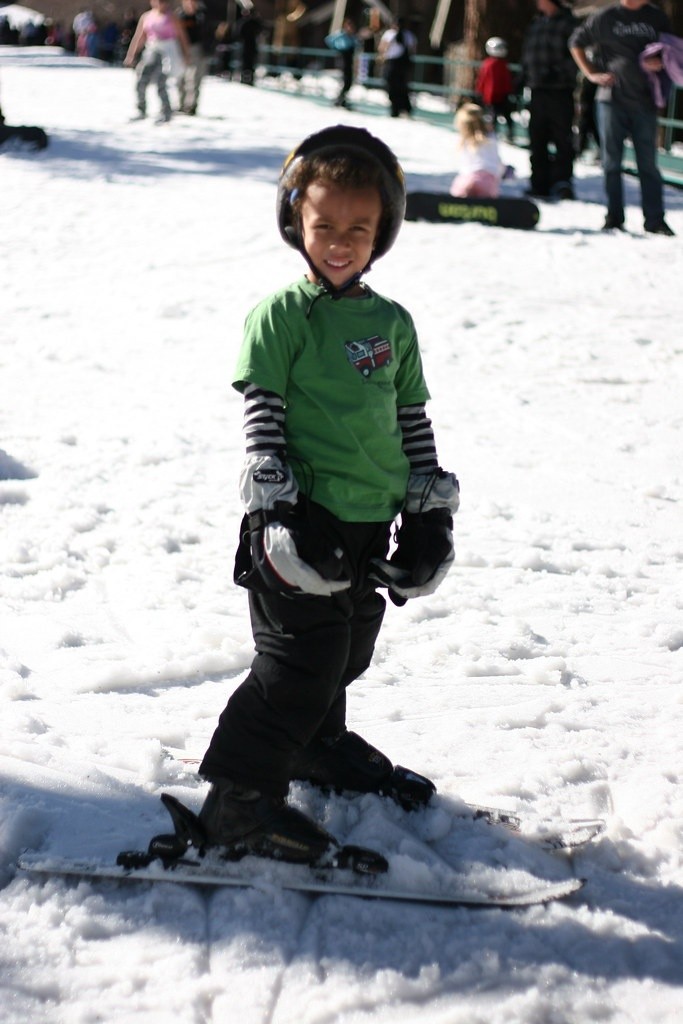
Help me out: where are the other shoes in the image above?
[644,219,674,235]
[603,218,622,231]
[289,730,435,813]
[196,784,388,881]
[155,114,170,123]
[131,110,148,121]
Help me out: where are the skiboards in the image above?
[14,771,604,906]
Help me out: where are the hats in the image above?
[486,36,508,56]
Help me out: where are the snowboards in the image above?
[402,189,541,233]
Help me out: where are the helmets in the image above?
[278,125,407,246]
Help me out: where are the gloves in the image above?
[366,469,460,608]
[240,452,351,600]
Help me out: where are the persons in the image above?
[378,15,417,118]
[523,0,581,201]
[566,0,673,236]
[123,0,193,123]
[174,0,215,115]
[0,0,141,65]
[237,8,264,85]
[200,124,461,873]
[324,18,358,111]
[451,103,506,202]
[476,38,524,139]
